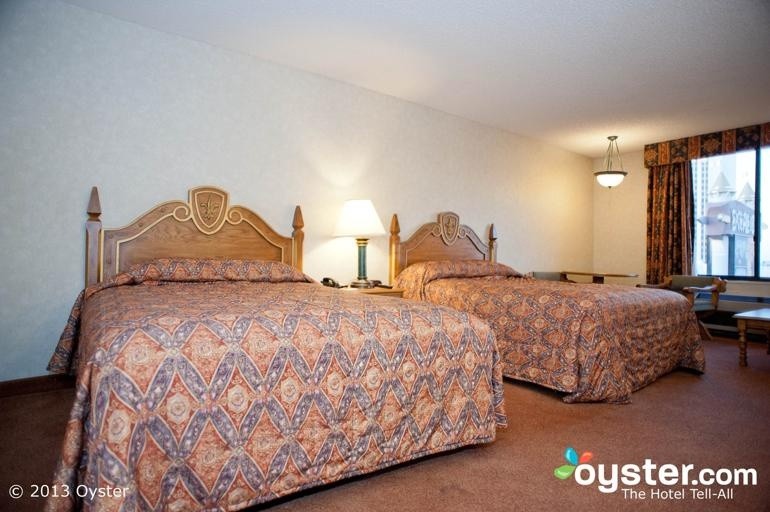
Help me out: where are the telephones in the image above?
[321,278,348,288]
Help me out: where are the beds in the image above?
[81,185,687,511]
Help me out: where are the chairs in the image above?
[633,274,727,344]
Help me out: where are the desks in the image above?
[733,306,770,367]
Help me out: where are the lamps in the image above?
[593,136,627,189]
